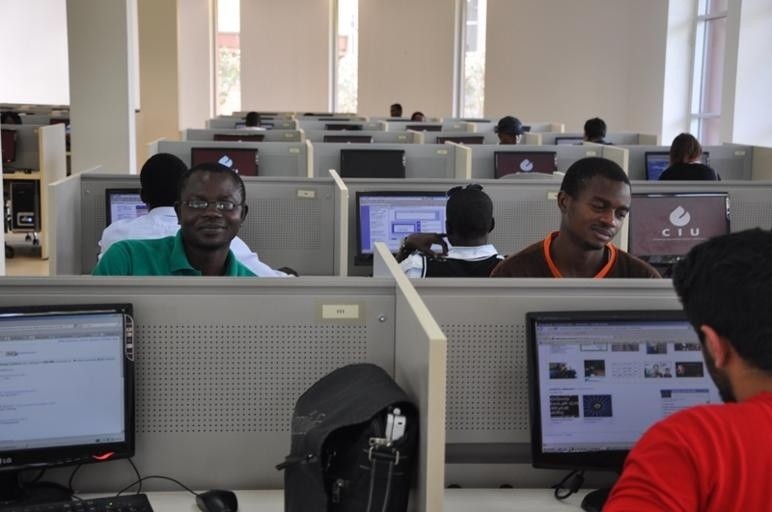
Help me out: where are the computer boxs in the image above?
[9,180,41,233]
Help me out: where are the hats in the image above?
[496,114,526,136]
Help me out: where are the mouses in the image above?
[196,489,238,512]
[23,168,31,173]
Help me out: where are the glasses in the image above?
[446,184,484,196]
[178,198,244,211]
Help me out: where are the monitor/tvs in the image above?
[645,151,710,180]
[191,147,259,176]
[213,134,265,142]
[627,191,731,278]
[105,188,149,227]
[555,137,584,145]
[356,191,448,266]
[323,135,372,143]
[405,124,441,131]
[325,124,363,131]
[436,136,484,144]
[339,148,406,178]
[1,129,17,173]
[0,303,136,507]
[526,310,725,512]
[494,151,558,179]
[234,122,274,129]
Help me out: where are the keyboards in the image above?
[0,494,153,512]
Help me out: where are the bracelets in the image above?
[401,234,413,255]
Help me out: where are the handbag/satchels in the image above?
[282,363,420,511]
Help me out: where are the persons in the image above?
[486,156,662,279]
[91,162,257,276]
[411,112,425,121]
[584,117,614,146]
[648,363,672,379]
[245,112,261,127]
[394,184,505,277]
[391,103,405,116]
[658,133,722,181]
[497,116,523,144]
[602,226,772,511]
[97,152,300,277]
